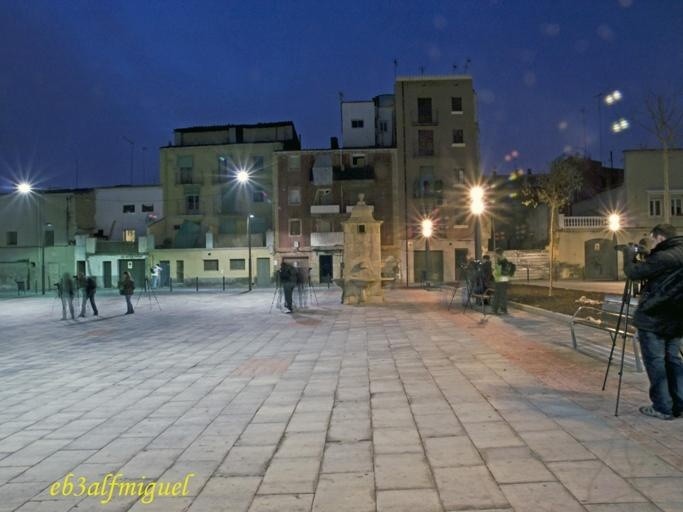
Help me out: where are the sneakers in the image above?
[639,405,674,420]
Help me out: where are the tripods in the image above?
[67,281,92,316]
[447,266,474,311]
[48,290,63,318]
[133,277,163,312]
[265,272,297,313]
[603,257,683,416]
[300,270,318,306]
[462,265,486,316]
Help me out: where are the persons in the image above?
[296,268,310,310]
[150,264,162,289]
[488,247,515,316]
[280,261,298,315]
[479,255,496,307]
[119,271,135,315]
[78,277,99,317]
[58,273,75,320]
[624,224,683,420]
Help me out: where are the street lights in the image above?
[237,169,253,292]
[607,212,623,284]
[418,218,435,285]
[16,182,48,295]
[468,186,485,259]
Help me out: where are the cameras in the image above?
[614,243,647,255]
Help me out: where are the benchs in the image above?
[471,281,511,315]
[568,294,643,373]
